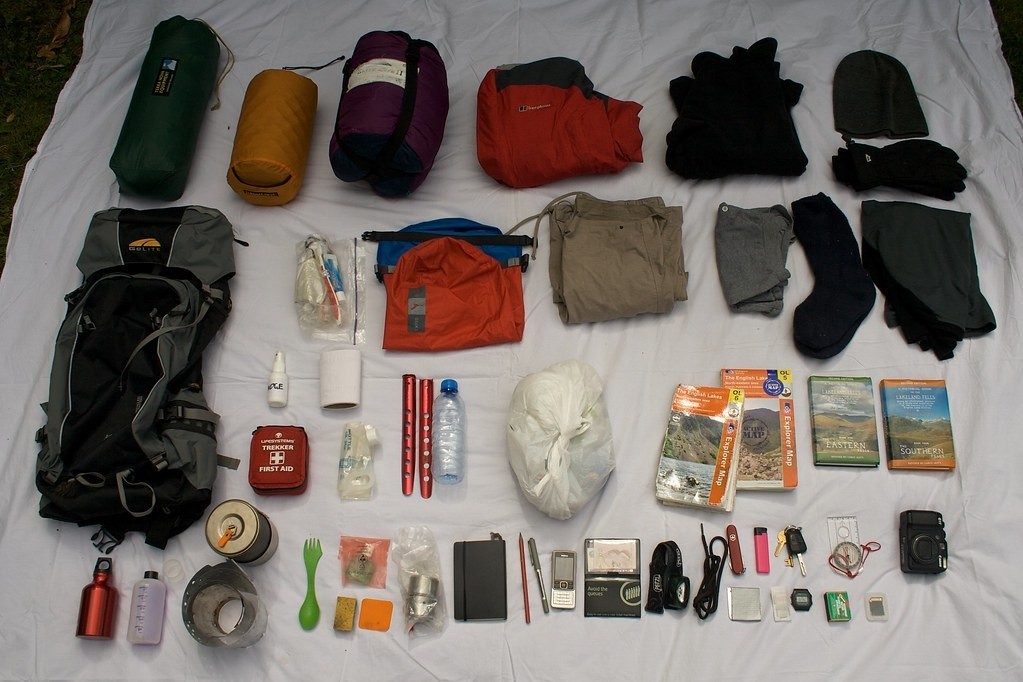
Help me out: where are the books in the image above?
[808,375,881,467]
[654,367,798,513]
[879,378,957,473]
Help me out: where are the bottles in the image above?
[127,571,166,644]
[76,557,117,638]
[431,379,465,484]
[267,351,288,407]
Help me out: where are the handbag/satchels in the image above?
[507,360,618,522]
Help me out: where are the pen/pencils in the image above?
[527,538,549,614]
[519,532,530,623]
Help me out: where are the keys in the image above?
[775,524,807,576]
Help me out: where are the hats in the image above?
[833,50,929,137]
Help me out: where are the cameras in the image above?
[900,509,948,575]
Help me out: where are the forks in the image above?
[299,539,323,630]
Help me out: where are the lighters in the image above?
[754,527,770,574]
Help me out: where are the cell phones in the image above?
[551,551,577,609]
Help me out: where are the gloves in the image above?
[831,139,967,201]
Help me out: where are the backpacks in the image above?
[33,207,247,549]
